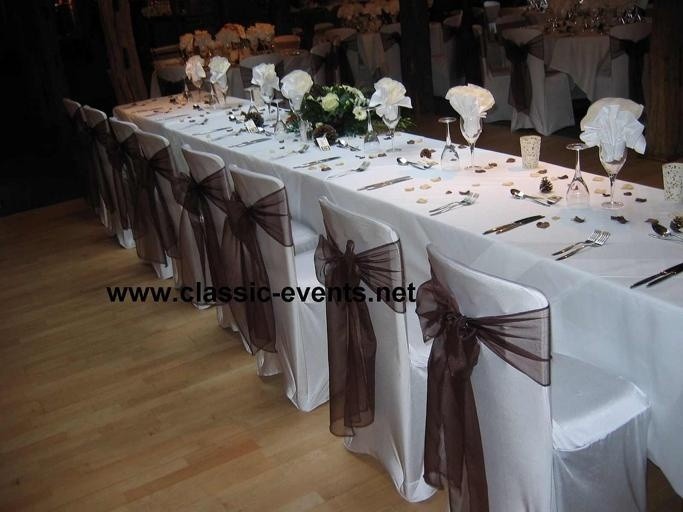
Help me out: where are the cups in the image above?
[361,105,380,157]
[260,88,274,122]
[565,143,590,209]
[180,74,193,107]
[245,88,259,114]
[207,80,220,111]
[439,117,461,171]
[271,98,289,150]
[379,106,402,154]
[459,118,482,171]
[519,134,542,169]
[662,162,682,202]
[288,97,306,133]
[599,143,627,209]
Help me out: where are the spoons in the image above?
[229,113,241,123]
[397,157,426,170]
[652,222,682,240]
[338,138,360,151]
[511,188,550,208]
[257,126,270,134]
[192,103,211,114]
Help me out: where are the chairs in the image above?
[328,29,380,87]
[502,29,576,135]
[180,143,317,310]
[431,10,463,95]
[239,54,287,92]
[109,117,136,248]
[134,129,185,280]
[610,24,651,104]
[384,24,405,82]
[421,245,653,512]
[310,43,329,88]
[316,194,442,502]
[458,27,513,125]
[62,98,83,204]
[84,106,109,226]
[229,163,330,413]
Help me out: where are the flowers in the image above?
[245,22,275,51]
[178,30,216,58]
[283,85,414,142]
[338,0,398,28]
[214,23,246,61]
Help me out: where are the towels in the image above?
[581,97,646,161]
[208,56,231,87]
[281,70,314,100]
[185,55,206,81]
[249,63,280,91]
[369,76,412,121]
[444,83,495,136]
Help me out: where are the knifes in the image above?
[358,175,413,195]
[482,215,544,237]
[631,260,683,299]
[230,137,271,150]
[294,156,340,169]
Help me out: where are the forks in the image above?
[429,194,472,214]
[274,145,309,161]
[430,193,480,229]
[327,161,370,181]
[552,229,601,255]
[180,118,208,129]
[556,230,610,263]
